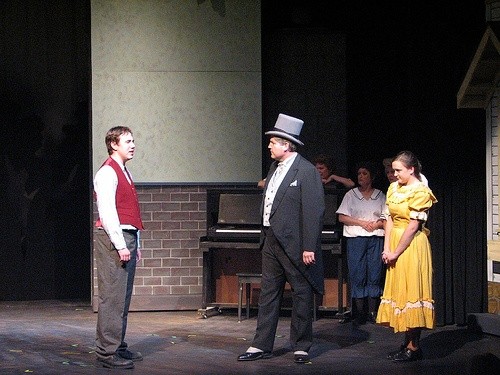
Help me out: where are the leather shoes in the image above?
[237,351,272,361]
[96,355,135,369]
[118,349,144,362]
[294,354,310,362]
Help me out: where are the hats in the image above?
[265,113,305,146]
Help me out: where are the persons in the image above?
[382,158,397,183]
[375,152,438,361]
[94,126,145,370]
[335,166,387,326]
[237,114,325,364]
[257,153,356,188]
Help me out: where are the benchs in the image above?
[236,272,317,322]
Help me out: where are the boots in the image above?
[369,296,380,323]
[351,297,368,323]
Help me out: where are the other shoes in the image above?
[388,346,406,359]
[393,348,422,360]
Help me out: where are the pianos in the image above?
[199,188,352,323]
